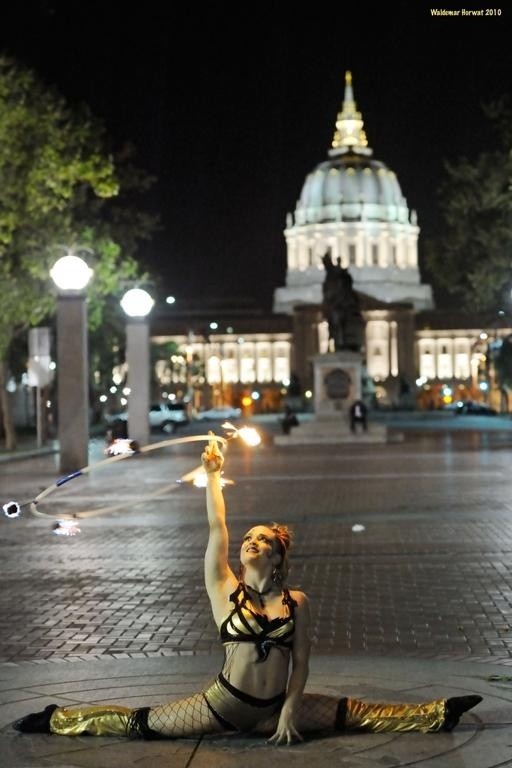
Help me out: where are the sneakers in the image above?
[437,693,484,734]
[12,703,60,736]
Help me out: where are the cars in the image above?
[440,397,499,418]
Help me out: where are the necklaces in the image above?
[244,583,274,610]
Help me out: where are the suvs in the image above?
[108,397,191,435]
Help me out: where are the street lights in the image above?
[116,287,156,450]
[47,251,96,476]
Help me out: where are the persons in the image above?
[11,426,483,749]
[317,247,358,292]
[278,404,301,434]
[347,396,372,434]
